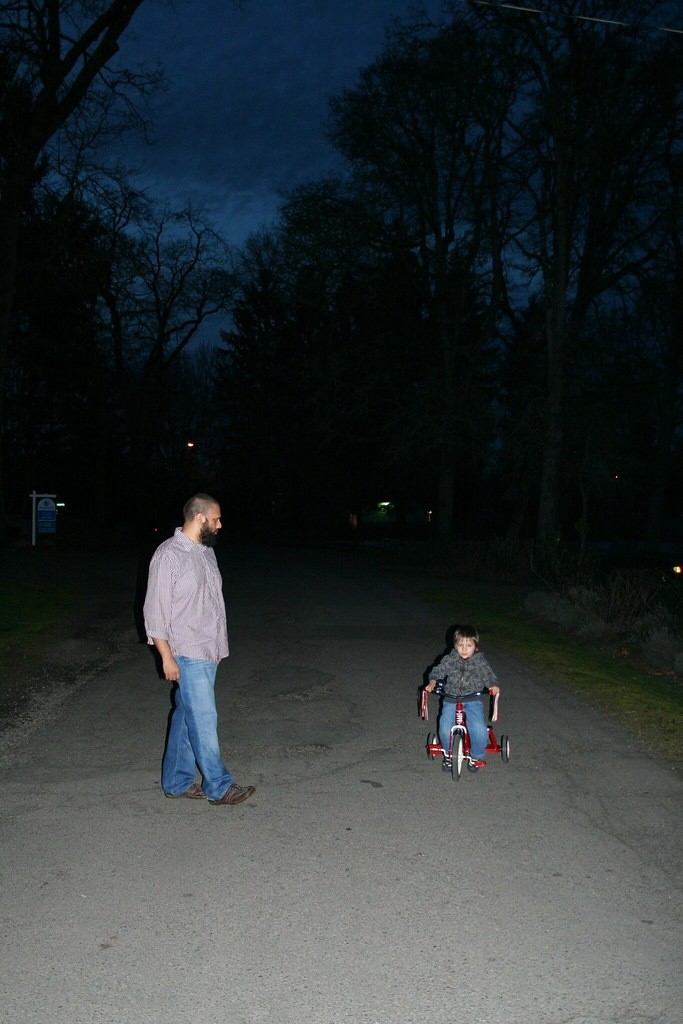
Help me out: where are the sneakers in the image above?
[442,756,453,772]
[208,782,255,805]
[164,782,207,799]
[467,759,480,772]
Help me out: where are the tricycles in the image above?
[420,679,511,782]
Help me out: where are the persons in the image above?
[424,625,501,772]
[143,494,256,805]
